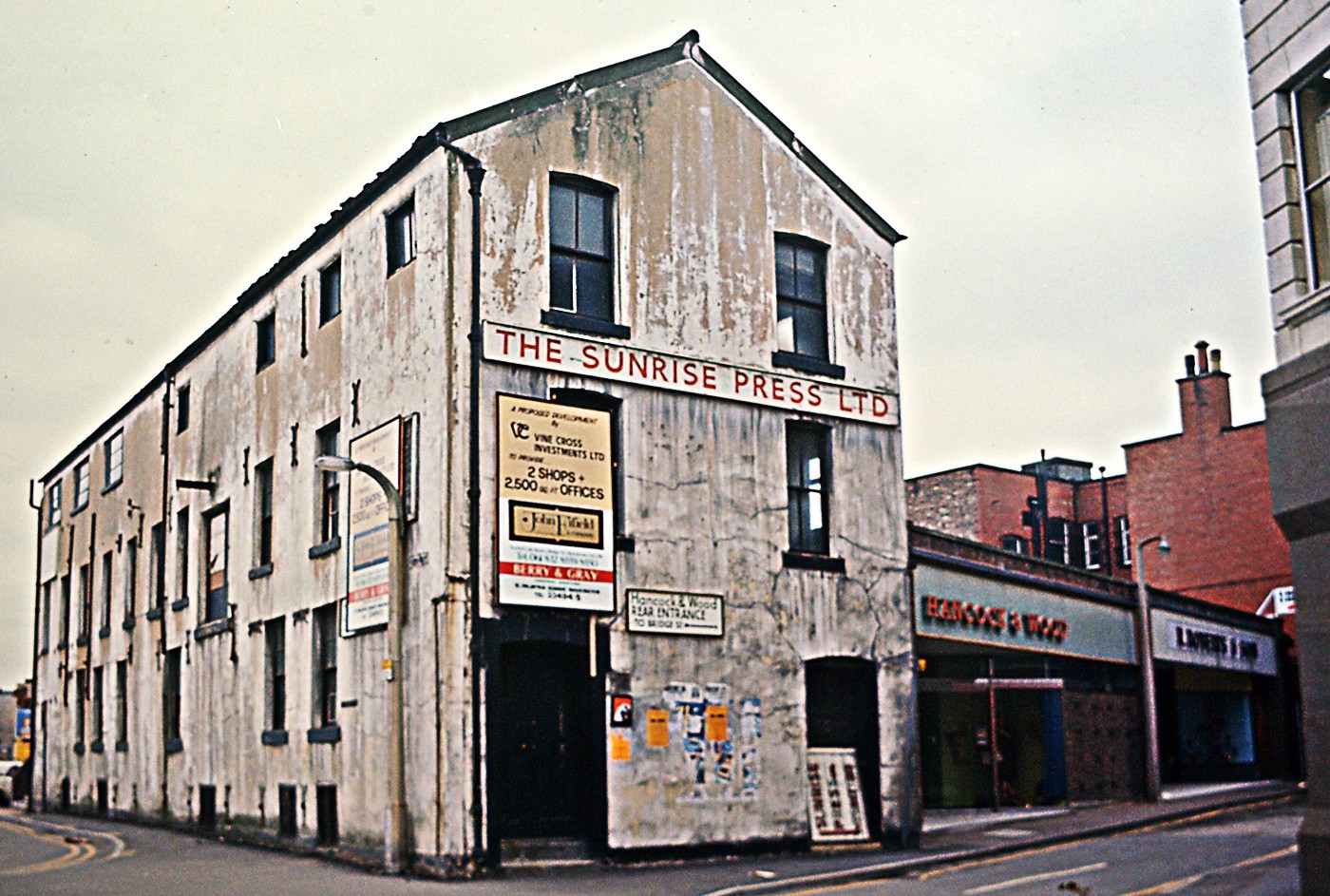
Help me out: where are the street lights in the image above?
[1136,534,1174,802]
[314,454,409,875]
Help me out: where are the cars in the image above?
[0,761,26,806]
